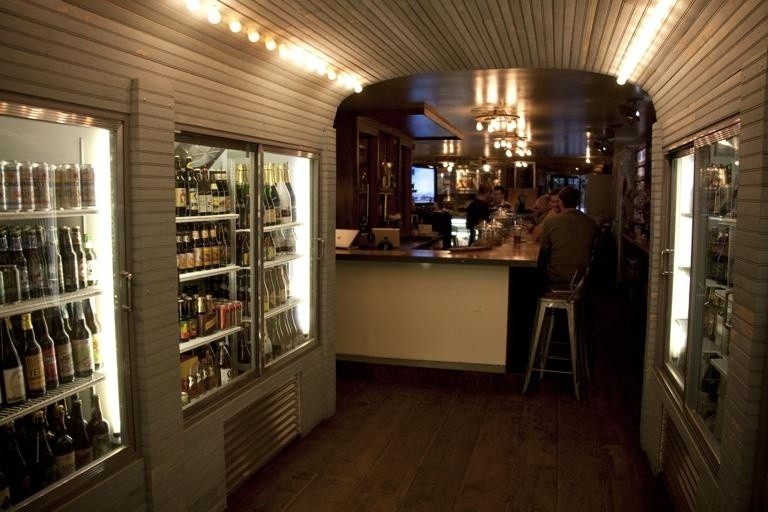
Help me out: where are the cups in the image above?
[511,225,523,246]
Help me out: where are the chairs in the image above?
[538,261,591,382]
[522,264,587,402]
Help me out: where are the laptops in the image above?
[370,227,400,248]
[335,229,360,250]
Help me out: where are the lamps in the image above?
[471,67,536,160]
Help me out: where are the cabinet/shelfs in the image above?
[350,117,414,228]
[687,120,742,439]
[176,130,322,424]
[0,91,141,509]
[659,136,696,402]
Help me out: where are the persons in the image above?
[466,184,492,247]
[487,185,513,223]
[532,193,550,214]
[532,186,598,285]
[538,190,561,223]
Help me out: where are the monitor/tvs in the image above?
[411,162,438,206]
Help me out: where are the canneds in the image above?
[212,298,243,329]
[1,160,97,212]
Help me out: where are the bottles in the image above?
[0,226,109,509]
[242,309,298,367]
[707,226,732,286]
[175,154,231,216]
[179,293,217,341]
[234,160,297,227]
[237,229,306,266]
[239,262,292,317]
[181,342,233,410]
[176,222,230,273]
[701,285,731,357]
[702,163,738,220]
